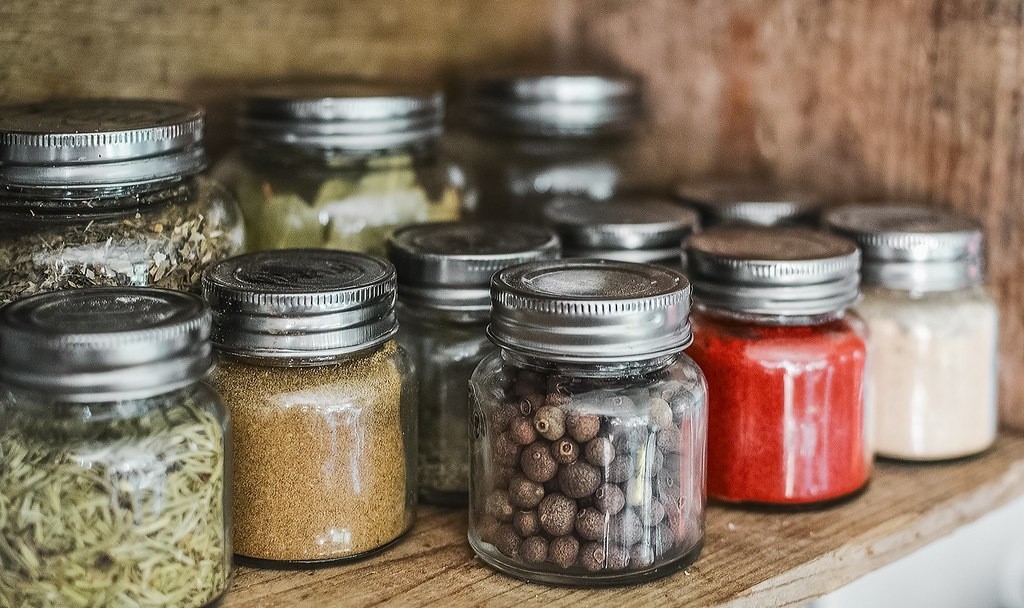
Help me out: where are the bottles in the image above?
[0,60,1003,608]
